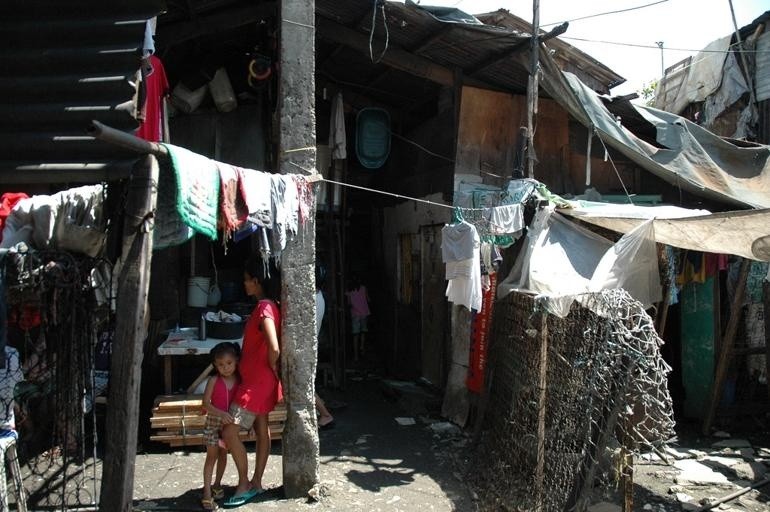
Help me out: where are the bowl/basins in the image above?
[204,315,250,339]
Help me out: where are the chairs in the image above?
[2,346,28,511]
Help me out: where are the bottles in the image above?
[209,69,237,115]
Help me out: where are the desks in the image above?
[155,326,244,396]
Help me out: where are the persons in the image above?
[221,256,283,506]
[0,321,28,435]
[314,265,334,431]
[345,271,370,364]
[200,342,242,511]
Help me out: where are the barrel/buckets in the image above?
[187,277,210,307]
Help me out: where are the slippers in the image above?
[199,493,219,511]
[212,484,225,500]
[318,421,336,432]
[223,487,266,507]
